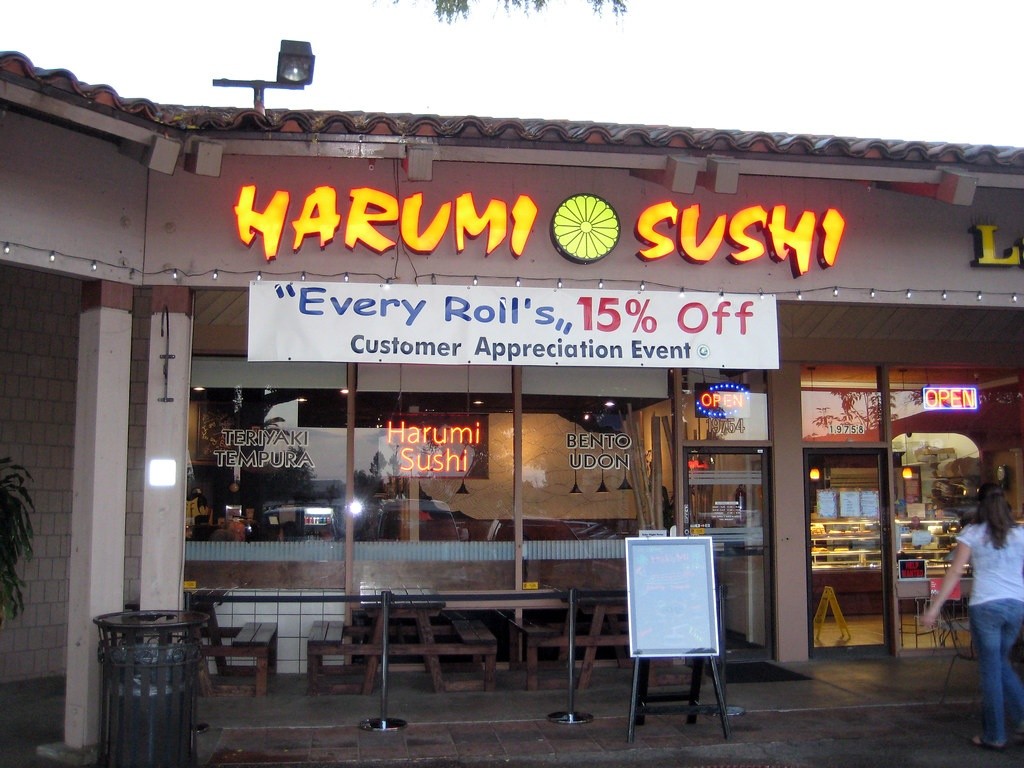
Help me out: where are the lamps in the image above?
[274,39,317,86]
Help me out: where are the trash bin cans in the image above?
[93,611,200,768]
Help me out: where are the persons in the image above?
[922,484,1024,750]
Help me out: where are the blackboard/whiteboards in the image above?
[622,535,722,658]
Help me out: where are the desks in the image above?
[561,580,638,692]
[306,620,351,693]
[176,579,243,696]
[361,584,452,691]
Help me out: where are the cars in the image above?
[486,516,602,587]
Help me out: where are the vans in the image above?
[371,497,462,541]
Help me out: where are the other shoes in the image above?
[971,736,1007,749]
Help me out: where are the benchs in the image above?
[498,608,562,688]
[231,620,277,694]
[451,616,501,690]
